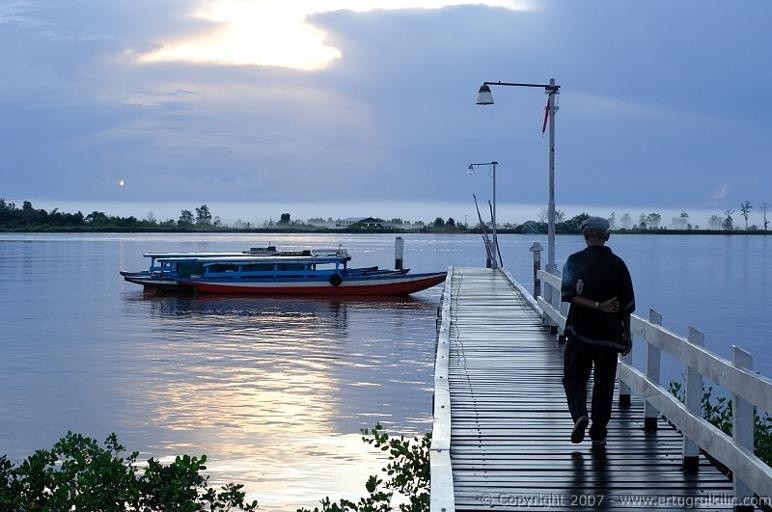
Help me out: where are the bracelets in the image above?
[594,300,600,310]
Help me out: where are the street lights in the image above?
[467,78,561,329]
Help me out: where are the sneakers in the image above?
[592,434,607,447]
[570,416,588,443]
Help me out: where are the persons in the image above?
[558,215,635,445]
